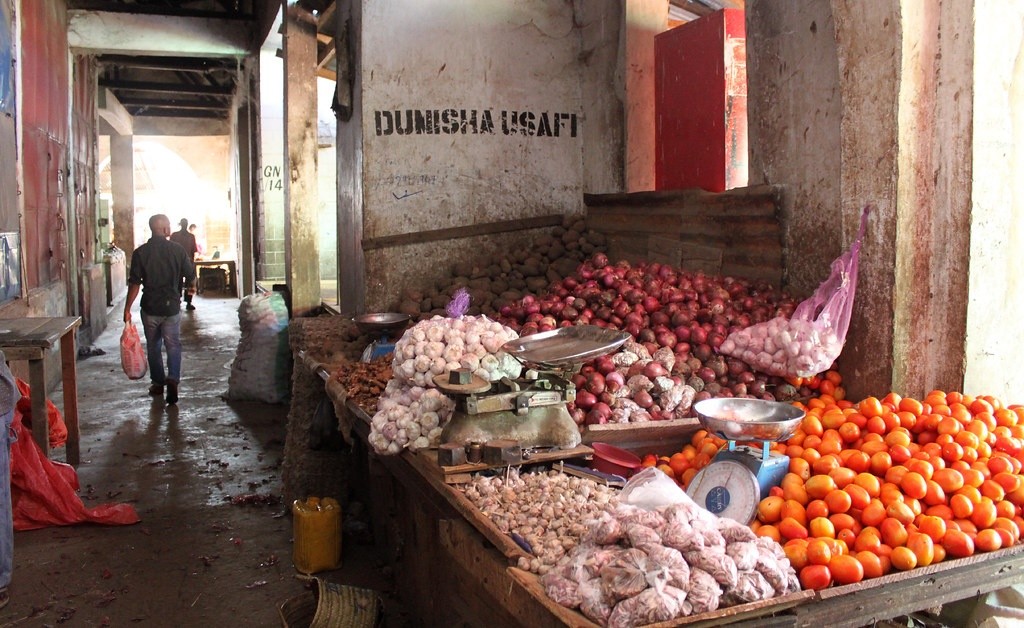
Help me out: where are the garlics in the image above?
[369,380,455,457]
[718,317,844,378]
[457,458,624,574]
[393,312,523,384]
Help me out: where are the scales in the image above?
[684,397,807,525]
[352,312,413,365]
[431,324,632,454]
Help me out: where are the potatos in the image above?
[397,213,608,334]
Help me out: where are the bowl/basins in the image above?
[351,312,412,334]
[693,397,806,440]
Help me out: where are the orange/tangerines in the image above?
[641,367,1024,592]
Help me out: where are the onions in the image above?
[567,343,839,426]
[492,252,806,349]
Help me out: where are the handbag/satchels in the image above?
[119,321,147,380]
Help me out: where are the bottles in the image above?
[292,497,343,574]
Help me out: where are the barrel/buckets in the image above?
[589,441,641,478]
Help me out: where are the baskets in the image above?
[277,573,383,627]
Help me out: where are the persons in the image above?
[170,218,197,310]
[212,245,220,260]
[188,224,196,233]
[123,214,195,404]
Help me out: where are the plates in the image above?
[501,323,632,369]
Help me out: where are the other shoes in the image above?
[167,379,179,404]
[148,385,164,394]
[186,301,195,310]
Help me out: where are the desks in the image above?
[0,316,84,463]
[103,255,127,305]
[195,261,235,295]
